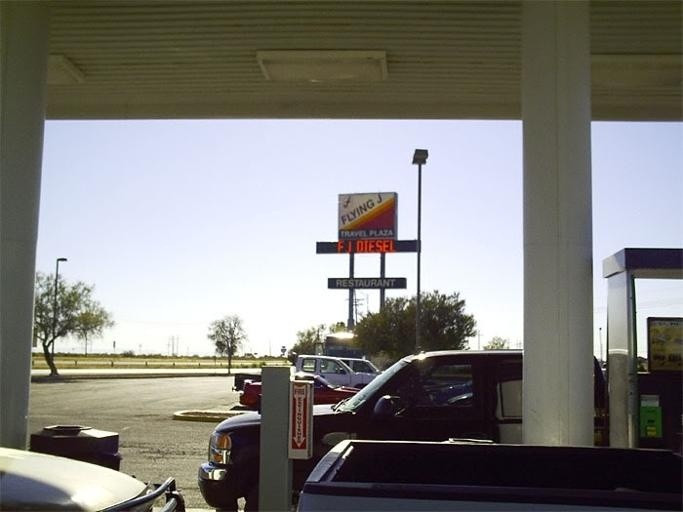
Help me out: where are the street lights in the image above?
[411,148,429,352]
[52,257,68,361]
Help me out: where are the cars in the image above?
[0,446,185,512]
[238,372,362,410]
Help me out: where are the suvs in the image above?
[198,349,609,511]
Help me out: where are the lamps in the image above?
[45,54,86,87]
[255,49,390,87]
[589,53,683,90]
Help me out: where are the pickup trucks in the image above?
[230,349,387,391]
[295,439,683,512]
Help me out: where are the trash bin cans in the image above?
[30,424,119,471]
